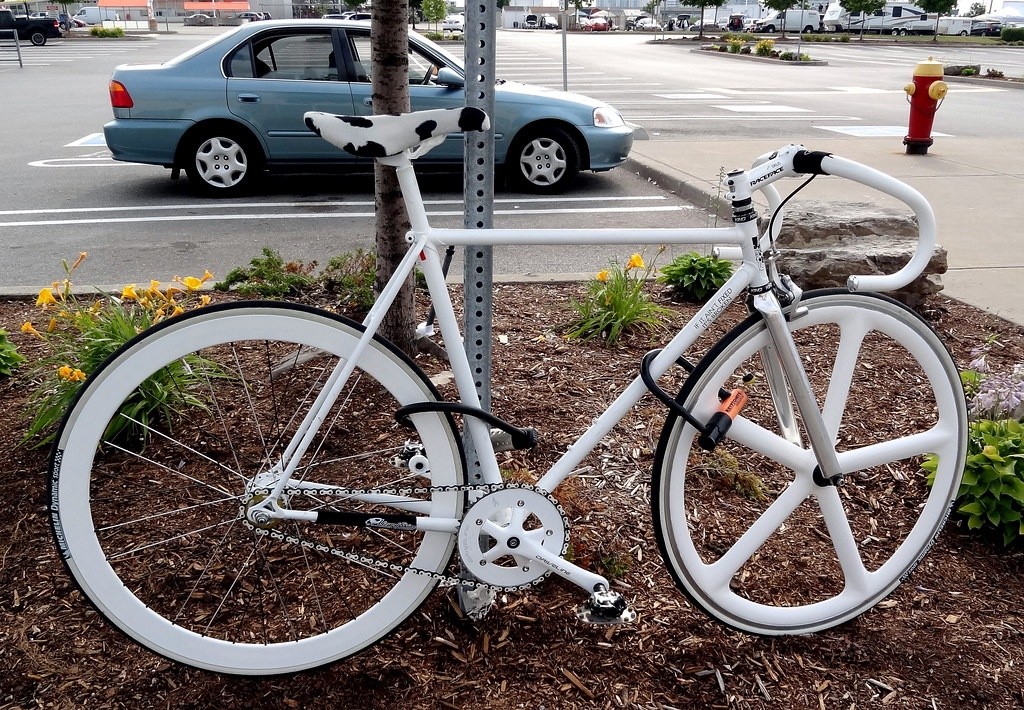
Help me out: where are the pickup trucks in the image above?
[0,10,63,47]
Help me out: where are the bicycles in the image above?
[45,108,971,676]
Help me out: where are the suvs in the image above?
[970,21,1003,37]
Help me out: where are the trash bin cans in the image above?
[149,18,157,31]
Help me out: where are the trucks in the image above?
[890,12,939,36]
[937,16,972,37]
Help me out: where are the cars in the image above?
[104,18,634,196]
[591,18,609,31]
[321,11,371,22]
[441,14,464,33]
[522,14,539,29]
[187,14,213,21]
[580,18,593,31]
[15,11,92,31]
[625,15,662,31]
[229,10,272,22]
[542,17,559,30]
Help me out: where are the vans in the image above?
[663,13,759,31]
[756,9,821,35]
[73,7,117,26]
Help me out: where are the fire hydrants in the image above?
[901,57,949,156]
